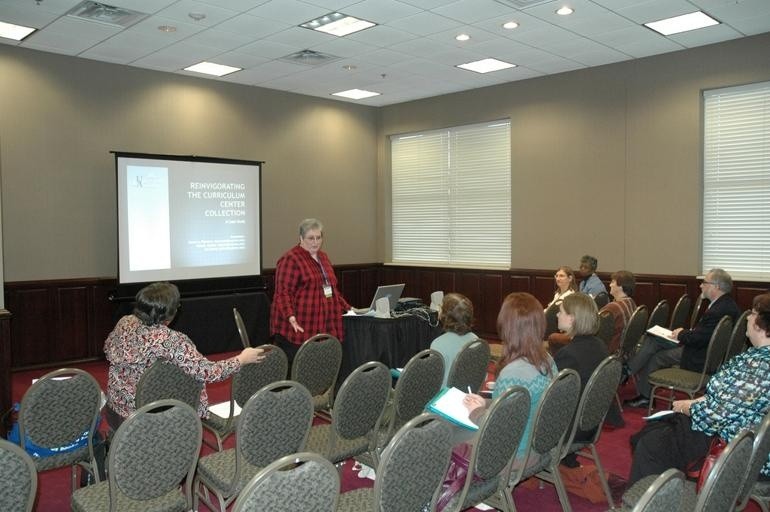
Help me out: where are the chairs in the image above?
[233,307,250,349]
[730,412,770,512]
[542,299,564,341]
[194,380,315,512]
[622,428,755,512]
[669,294,692,330]
[352,349,446,470]
[610,467,697,512]
[233,452,341,512]
[72,399,203,511]
[690,292,704,330]
[202,343,289,452]
[105,358,202,456]
[447,338,491,395]
[7,366,102,494]
[504,367,582,511]
[560,353,621,512]
[0,438,38,512]
[290,333,344,412]
[725,309,753,367]
[595,291,609,310]
[597,309,616,345]
[301,361,392,474]
[337,411,454,511]
[610,305,649,412]
[647,314,734,416]
[648,300,671,330]
[436,384,531,512]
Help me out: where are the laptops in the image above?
[352,283,405,314]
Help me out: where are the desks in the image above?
[336,306,441,395]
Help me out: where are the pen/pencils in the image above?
[467,386,472,395]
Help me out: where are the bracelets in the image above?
[681,407,684,415]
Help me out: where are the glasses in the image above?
[747,310,758,317]
[554,275,566,279]
[701,281,714,286]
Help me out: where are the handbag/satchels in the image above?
[695,436,727,493]
[435,442,480,511]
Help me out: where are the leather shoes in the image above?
[619,369,628,386]
[623,395,657,410]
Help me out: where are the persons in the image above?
[103,282,266,430]
[598,270,637,354]
[269,217,358,391]
[579,254,609,299]
[544,266,576,343]
[553,292,610,467]
[423,292,559,470]
[430,292,480,392]
[619,268,739,411]
[625,294,770,490]
